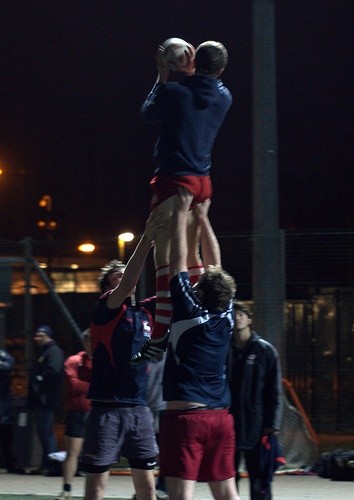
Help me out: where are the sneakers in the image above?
[129,332,170,364]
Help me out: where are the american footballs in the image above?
[161,38,192,70]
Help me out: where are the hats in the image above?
[37,326,54,339]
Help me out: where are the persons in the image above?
[158,184,242,500]
[226,302,283,500]
[79,209,165,495]
[54,328,93,499]
[29,325,64,472]
[130,41,234,365]
[1,349,22,473]
